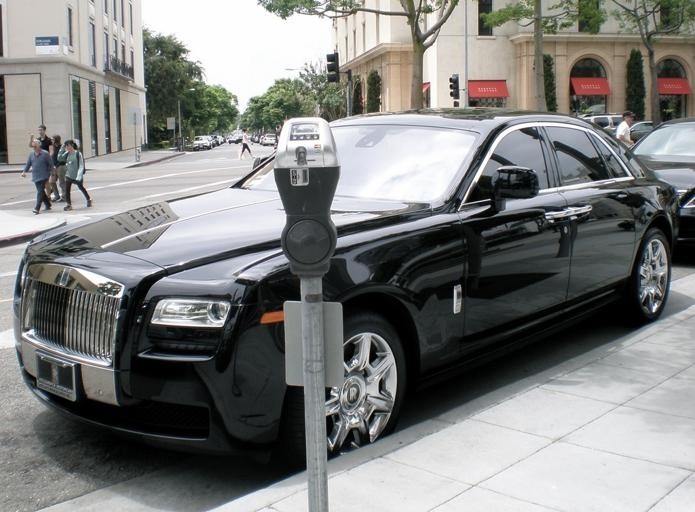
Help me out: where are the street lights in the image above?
[176,88,196,139]
[284,66,306,75]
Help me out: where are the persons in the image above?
[50,135,68,202]
[28,124,55,201]
[240,128,255,159]
[57,140,93,211]
[21,139,53,212]
[615,110,636,148]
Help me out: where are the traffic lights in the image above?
[326,54,339,83]
[449,74,459,98]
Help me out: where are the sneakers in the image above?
[52,196,66,203]
[87,199,92,207]
[64,205,72,211]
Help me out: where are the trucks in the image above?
[387,205,595,288]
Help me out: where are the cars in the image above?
[192,136,226,150]
[628,120,664,143]
[228,134,243,144]
[629,117,695,269]
[13,106,683,469]
[247,134,276,146]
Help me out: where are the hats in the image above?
[623,111,635,116]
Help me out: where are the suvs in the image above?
[576,112,634,138]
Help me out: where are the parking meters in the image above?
[274,116,345,512]
